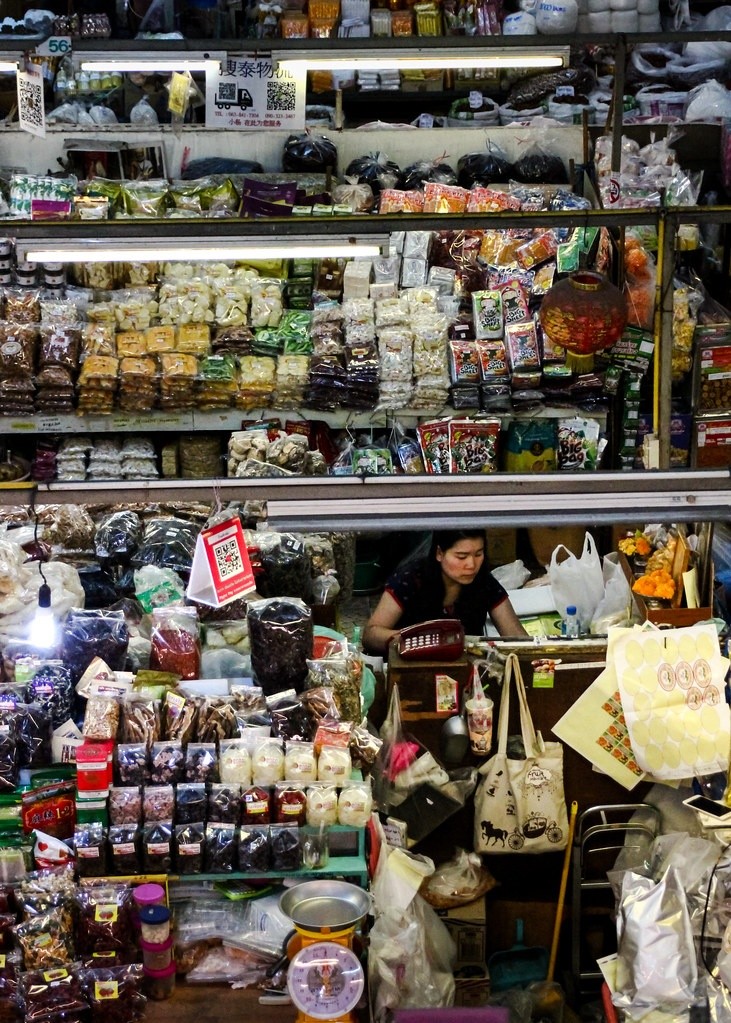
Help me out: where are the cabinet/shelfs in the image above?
[80,625,368,889]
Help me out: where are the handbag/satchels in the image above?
[589,549,643,634]
[370,684,479,850]
[551,623,731,791]
[549,531,605,634]
[615,624,731,780]
[474,653,572,855]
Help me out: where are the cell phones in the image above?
[683,794,731,821]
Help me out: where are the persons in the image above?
[362,529,530,652]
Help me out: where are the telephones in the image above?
[397,618,465,662]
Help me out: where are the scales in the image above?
[278,879,372,1023]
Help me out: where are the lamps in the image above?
[75,44,572,72]
[14,234,390,259]
[265,490,730,532]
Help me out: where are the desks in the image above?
[139,973,299,1023]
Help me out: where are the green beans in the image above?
[198,351,235,381]
[253,312,312,356]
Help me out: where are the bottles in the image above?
[562,606,581,637]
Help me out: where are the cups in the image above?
[465,698,493,755]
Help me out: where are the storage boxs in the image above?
[434,897,491,1007]
